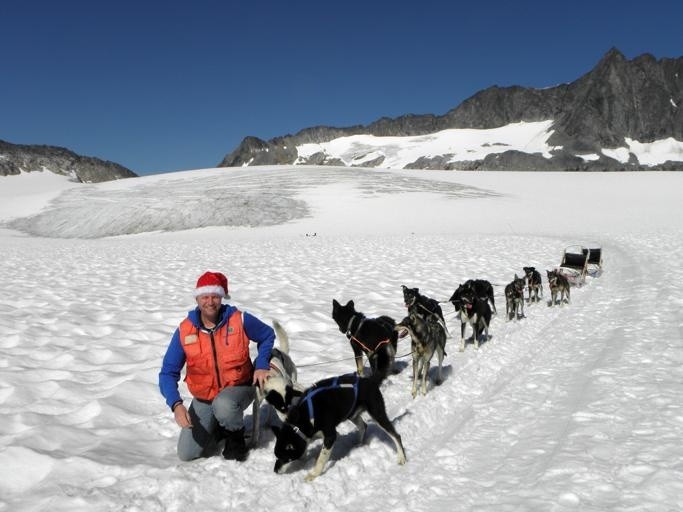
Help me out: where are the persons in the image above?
[158,272,276,461]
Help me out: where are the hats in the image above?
[193,272,231,299]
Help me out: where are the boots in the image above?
[223,427,247,461]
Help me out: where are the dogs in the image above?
[332,299,398,378]
[249,319,304,450]
[268,349,406,483]
[505,274,527,321]
[393,285,447,399]
[546,269,571,307]
[523,267,543,307]
[448,279,496,352]
